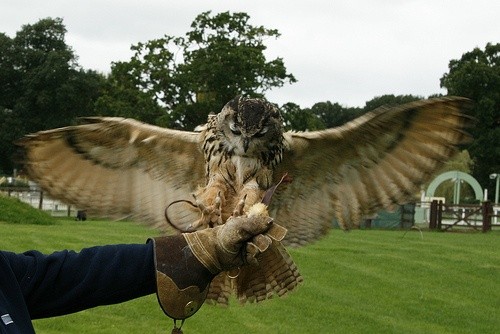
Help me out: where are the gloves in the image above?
[145,216,271,334]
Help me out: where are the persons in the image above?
[0,217,272,334]
